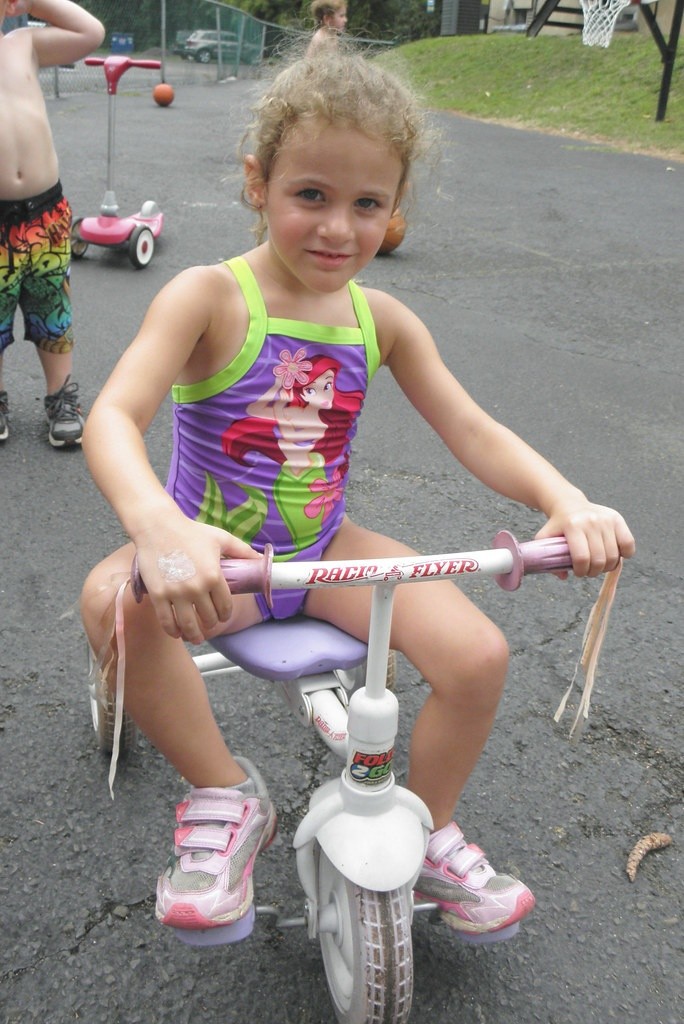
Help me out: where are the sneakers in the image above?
[44,374,85,446]
[153,756,278,929]
[413,820,535,934]
[0,391,10,440]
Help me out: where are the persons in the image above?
[0,1,106,451]
[76,47,637,935]
[308,1,356,55]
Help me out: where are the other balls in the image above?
[368,207,407,255]
[151,83,176,108]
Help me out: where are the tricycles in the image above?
[87,525,578,1024]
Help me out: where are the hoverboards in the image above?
[66,58,163,270]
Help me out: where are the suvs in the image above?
[182,29,259,65]
